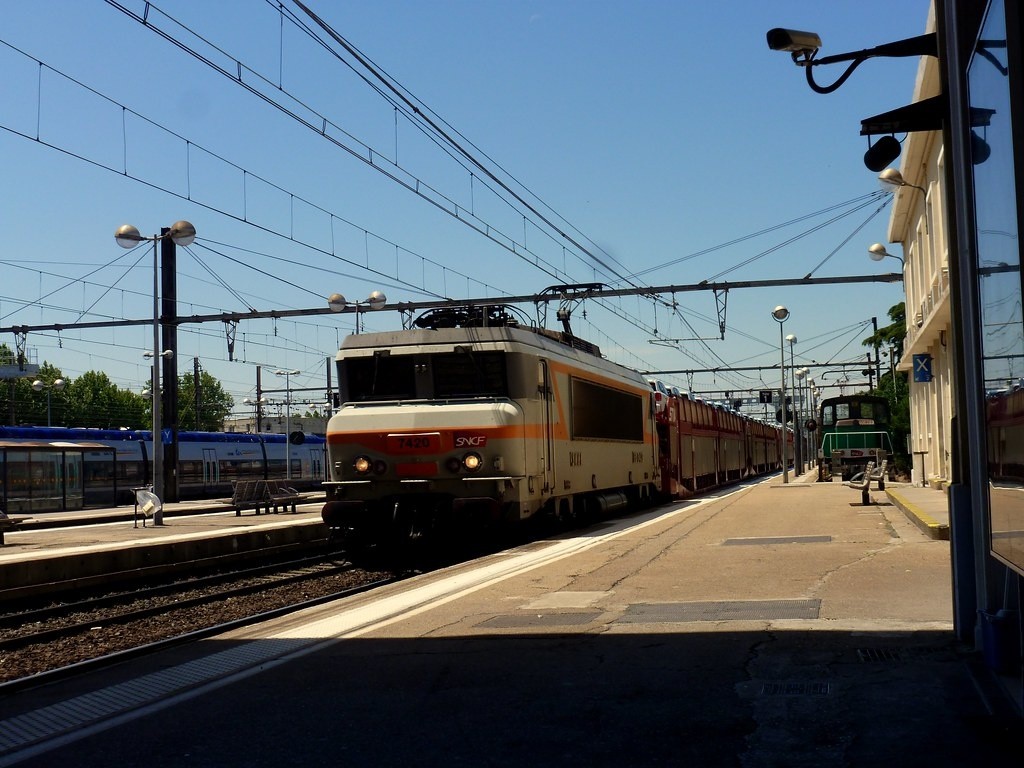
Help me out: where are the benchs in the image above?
[842,461,875,506]
[869,460,888,490]
[0,511,33,544]
[216,481,313,516]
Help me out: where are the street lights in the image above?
[327,290,388,336]
[274,369,300,480]
[242,397,268,434]
[309,403,333,434]
[794,366,820,474]
[786,335,798,477]
[771,306,791,484]
[31,378,66,428]
[113,220,197,527]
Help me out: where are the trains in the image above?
[0,426,328,505]
[321,327,807,555]
[985,378,1024,484]
[820,391,891,473]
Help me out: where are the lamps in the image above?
[869,244,906,291]
[879,168,928,233]
